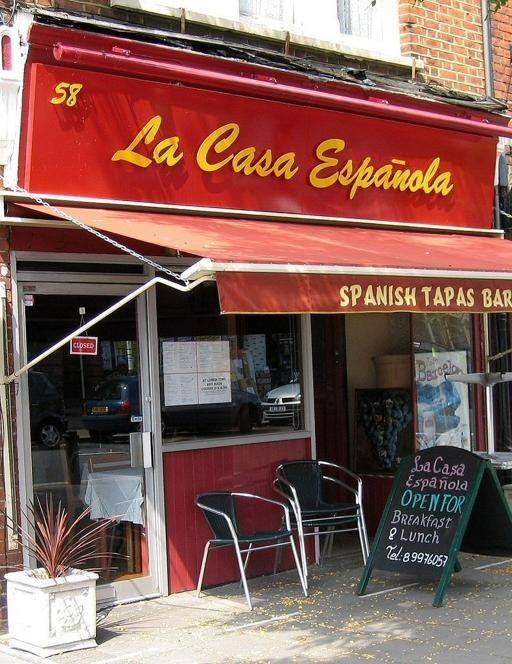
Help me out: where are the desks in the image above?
[27,446,143,572]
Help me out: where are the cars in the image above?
[259,372,303,424]
[82,370,264,437]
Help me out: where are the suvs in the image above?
[26,369,69,449]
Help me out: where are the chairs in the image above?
[194,460,372,614]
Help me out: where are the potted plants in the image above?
[7,489,120,658]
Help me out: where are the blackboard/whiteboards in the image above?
[372,446,487,576]
